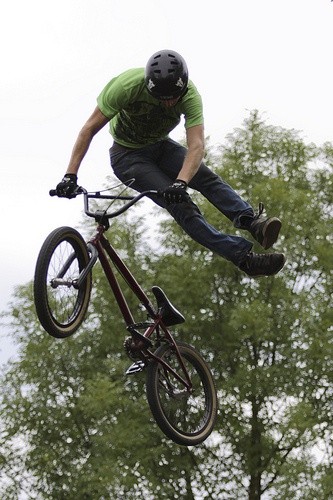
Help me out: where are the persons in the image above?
[55,48,287,281]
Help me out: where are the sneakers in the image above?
[244,252,286,278]
[248,202,282,250]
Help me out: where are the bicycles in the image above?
[32,178,219,447]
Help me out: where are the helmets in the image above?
[144,50,188,100]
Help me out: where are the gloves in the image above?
[57,173,78,199]
[162,179,187,203]
